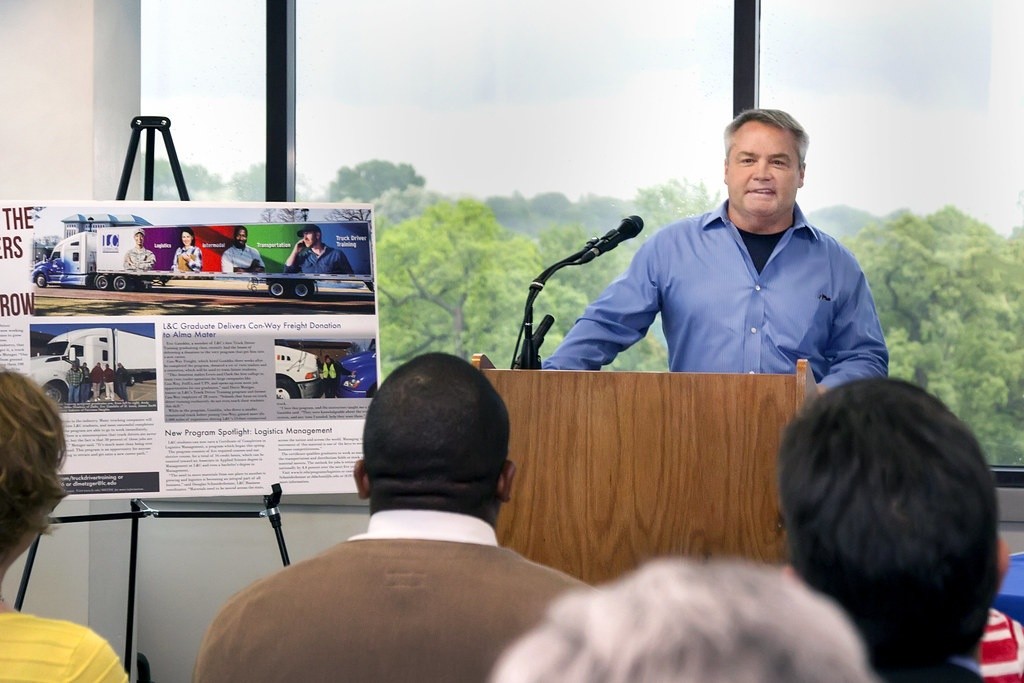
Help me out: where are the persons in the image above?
[116,362,129,401]
[66,363,116,402]
[777,376,1012,683]
[221,226,266,273]
[124,228,156,271]
[171,227,203,272]
[544,108,894,393]
[315,355,356,398]
[0,369,131,682]
[283,224,355,274]
[193,351,596,682]
[489,555,878,682]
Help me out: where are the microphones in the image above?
[578,216,644,264]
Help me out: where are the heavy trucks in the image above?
[30,222,373,300]
[30,328,157,404]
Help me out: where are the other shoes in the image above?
[110,399,114,401]
[86,400,91,402]
[105,396,109,400]
[94,398,97,402]
[97,398,100,401]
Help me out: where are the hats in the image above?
[297,224,321,237]
[133,228,145,238]
